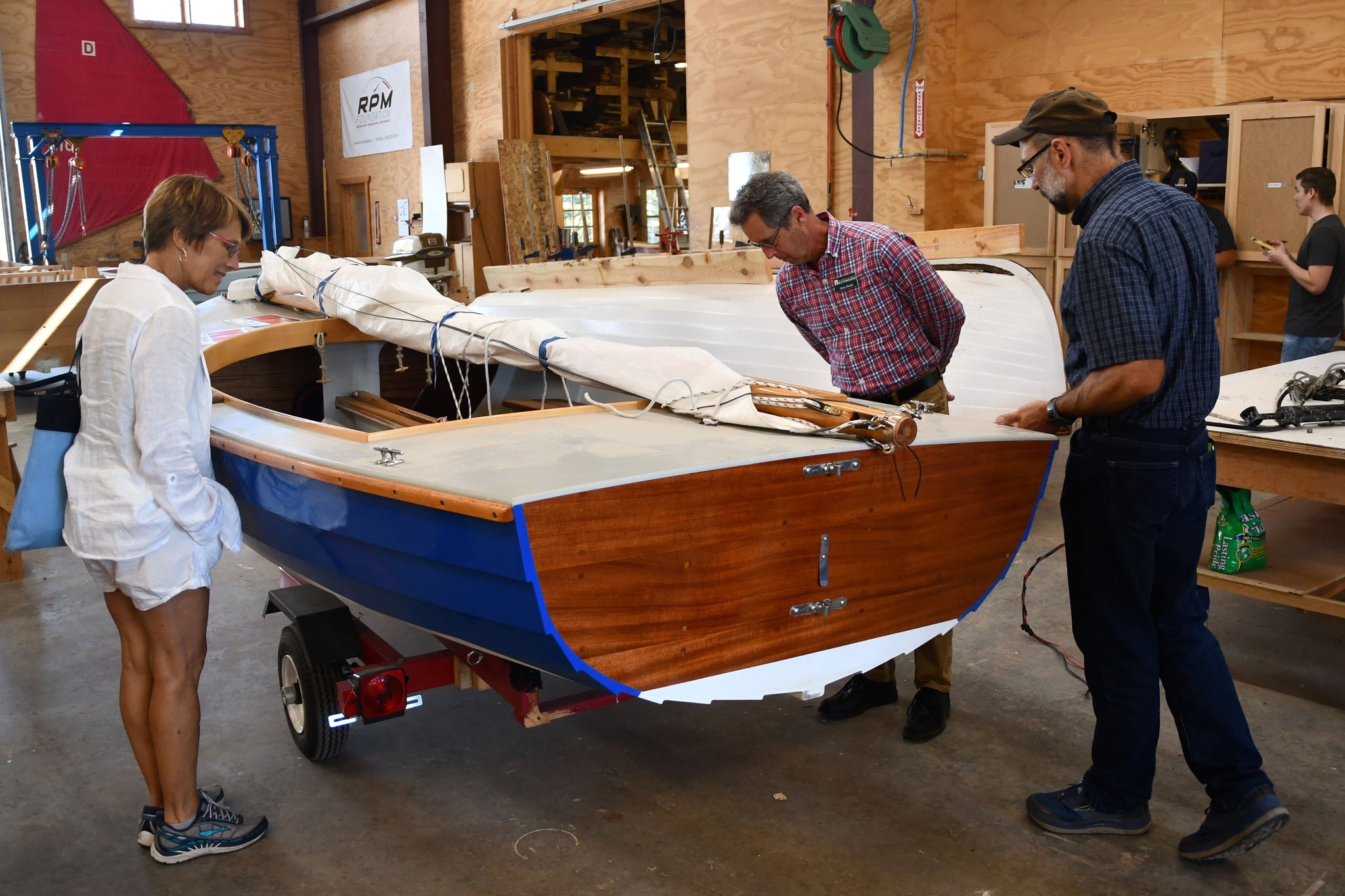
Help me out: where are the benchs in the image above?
[502,398,589,411]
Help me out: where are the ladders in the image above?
[636,108,690,250]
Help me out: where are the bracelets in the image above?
[1046,396,1080,428]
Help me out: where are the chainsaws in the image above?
[822,1,890,75]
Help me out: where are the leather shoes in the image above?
[903,686,951,742]
[818,673,899,720]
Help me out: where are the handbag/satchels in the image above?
[2,336,82,553]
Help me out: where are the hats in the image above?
[1169,170,1197,196]
[992,87,1117,148]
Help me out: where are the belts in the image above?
[1083,417,1206,446]
[840,368,941,406]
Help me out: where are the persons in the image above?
[731,171,958,745]
[61,173,272,863]
[1168,168,1238,270]
[1263,166,1345,362]
[994,82,1291,859]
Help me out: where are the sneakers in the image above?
[1025,782,1152,835]
[150,788,268,864]
[137,784,224,847]
[1178,770,1290,860]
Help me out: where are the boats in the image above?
[180,269,1063,704]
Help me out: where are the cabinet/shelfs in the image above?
[986,102,1345,377]
[1189,351,1345,619]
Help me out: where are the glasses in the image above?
[1017,142,1070,178]
[207,230,240,258]
[747,205,798,249]
